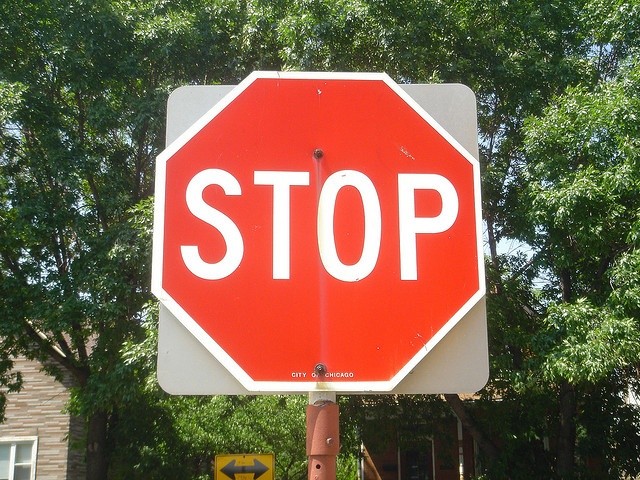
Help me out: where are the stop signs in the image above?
[152,72,490,395]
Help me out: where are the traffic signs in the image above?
[216,456,274,480]
